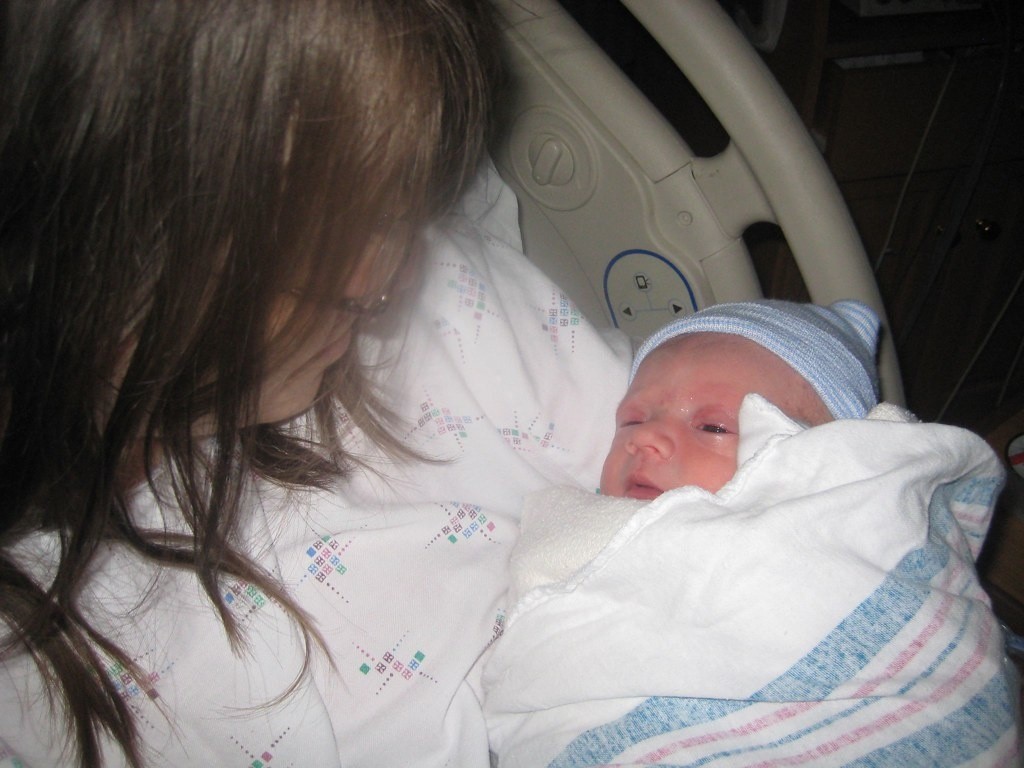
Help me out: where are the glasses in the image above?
[194,209,414,315]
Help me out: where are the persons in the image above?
[0,0,650,768]
[482,301,1024,768]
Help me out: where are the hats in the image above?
[628,299,881,419]
